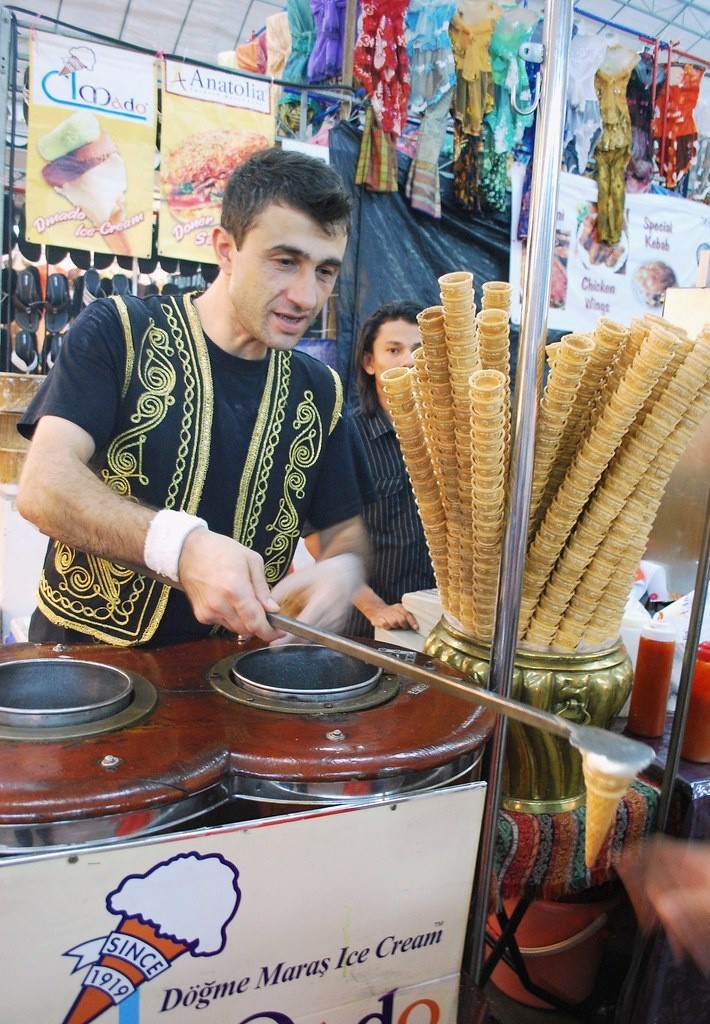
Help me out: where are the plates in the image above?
[577,213,629,273]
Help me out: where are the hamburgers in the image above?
[159,130,268,227]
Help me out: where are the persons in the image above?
[620,834,710,981]
[301,302,438,639]
[17,147,379,645]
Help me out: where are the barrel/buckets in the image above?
[231,641,384,703]
[0,656,135,729]
[483,894,621,1009]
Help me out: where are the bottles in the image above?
[627,611,676,738]
[680,641,710,763]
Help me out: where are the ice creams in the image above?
[36,108,132,256]
[583,750,656,869]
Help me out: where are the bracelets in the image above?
[144,508,209,583]
